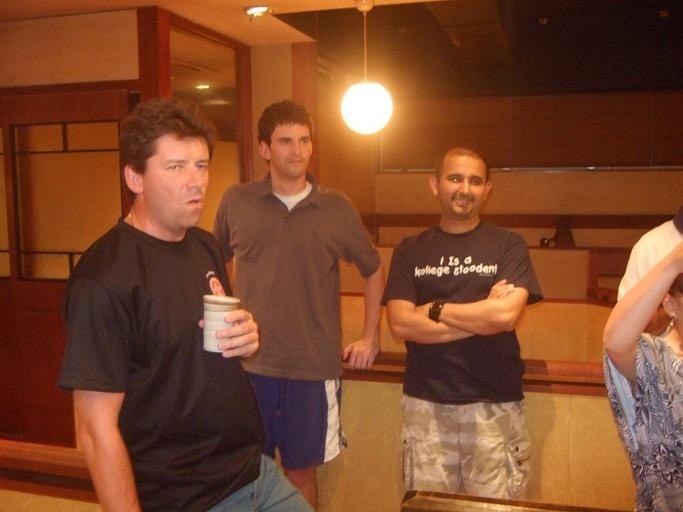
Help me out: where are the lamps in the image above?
[340,0,393,135]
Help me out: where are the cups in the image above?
[204,295,241,353]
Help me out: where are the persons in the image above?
[612,204,683,338]
[378,142,545,506]
[209,97,386,509]
[54,90,321,511]
[599,237,683,512]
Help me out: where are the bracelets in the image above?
[426,297,444,323]
